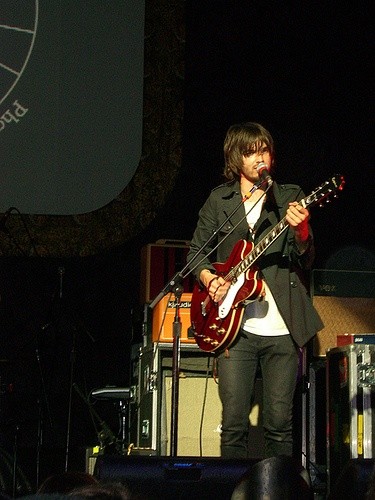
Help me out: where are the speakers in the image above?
[91,454,263,500]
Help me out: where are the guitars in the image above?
[190,172,346,354]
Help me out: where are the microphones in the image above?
[0,210,9,232]
[257,163,273,184]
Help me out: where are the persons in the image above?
[185,122,325,456]
[327,459,375,500]
[0,449,132,500]
